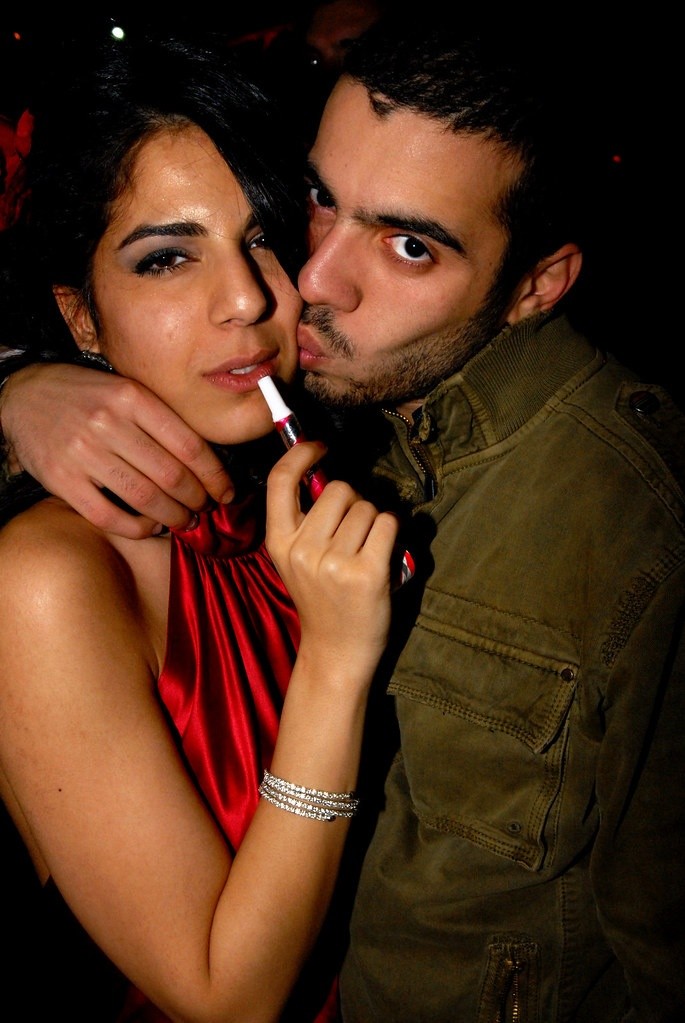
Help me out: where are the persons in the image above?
[0,22,685,1023]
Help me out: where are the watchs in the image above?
[0,348,62,388]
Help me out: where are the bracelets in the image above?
[257,768,360,822]
